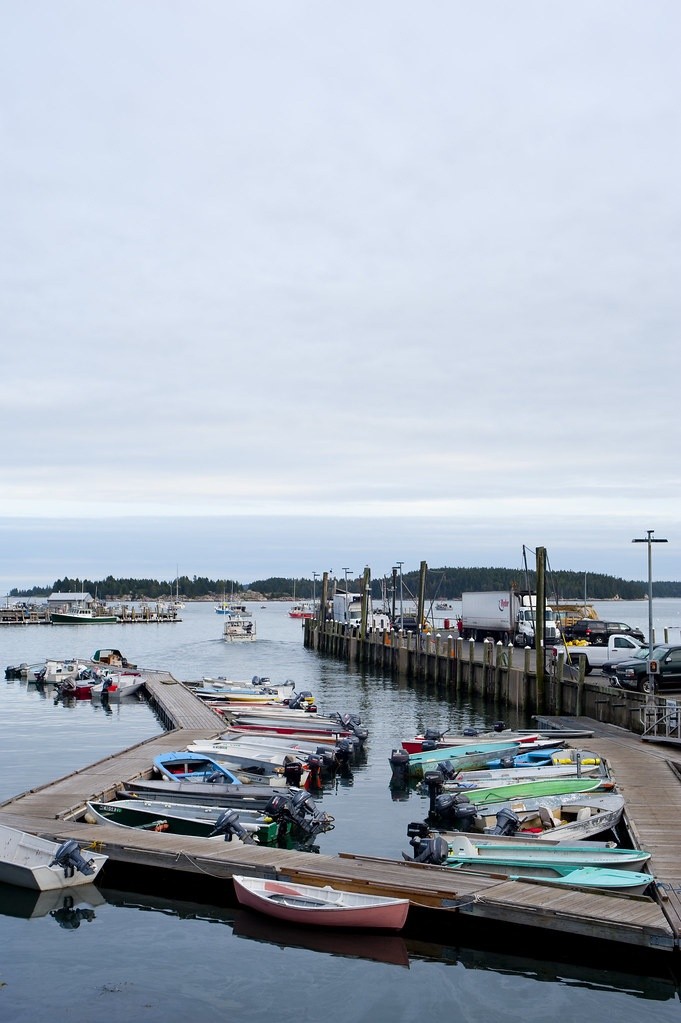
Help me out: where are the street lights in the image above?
[312,571,321,618]
[631,530,668,695]
[396,562,405,629]
[342,568,353,621]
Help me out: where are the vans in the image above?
[572,620,645,644]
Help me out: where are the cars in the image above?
[602,643,681,695]
[392,617,417,634]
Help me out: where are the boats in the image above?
[168,563,185,609]
[51,608,117,625]
[222,609,257,643]
[435,603,453,611]
[4,648,146,698]
[232,874,409,931]
[86,676,369,844]
[0,825,109,891]
[215,601,245,614]
[389,722,657,896]
[289,601,314,619]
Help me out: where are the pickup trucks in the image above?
[552,634,650,675]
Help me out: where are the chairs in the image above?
[577,807,592,821]
[452,837,479,856]
[539,806,561,829]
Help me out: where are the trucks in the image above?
[333,593,371,628]
[462,591,562,648]
